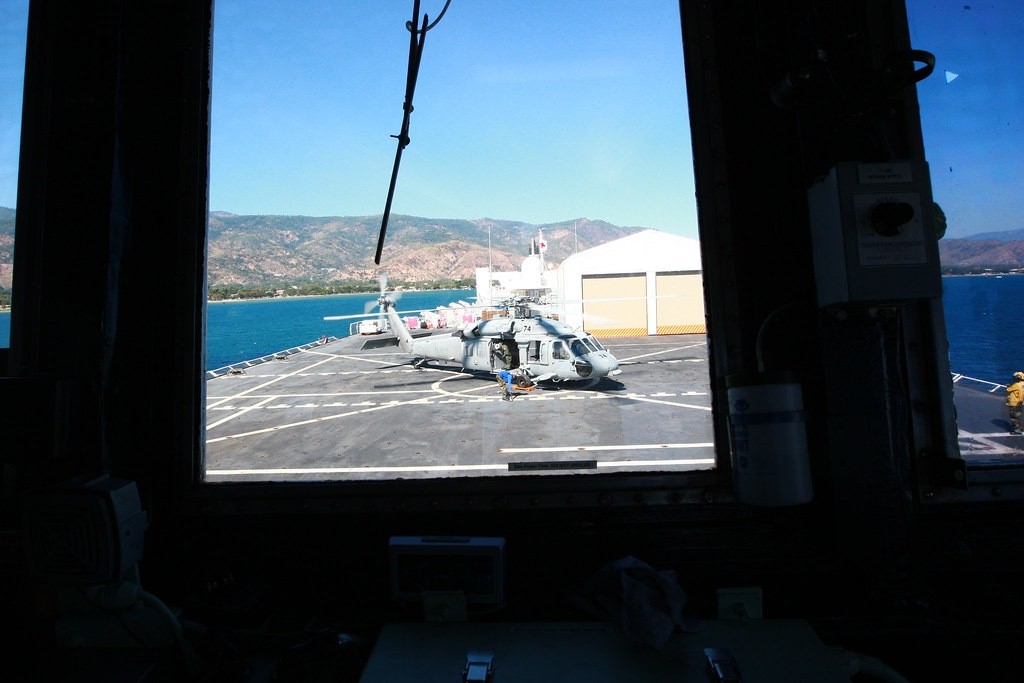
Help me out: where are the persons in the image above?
[496,371,517,402]
[1005,372,1024,435]
[502,344,512,370]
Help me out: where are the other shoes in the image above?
[1010,431,1023,435]
[503,397,510,401]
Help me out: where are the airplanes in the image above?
[322,272,623,389]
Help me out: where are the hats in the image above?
[1013,372,1024,379]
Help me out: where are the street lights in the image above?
[570,218,578,254]
[487,224,492,272]
